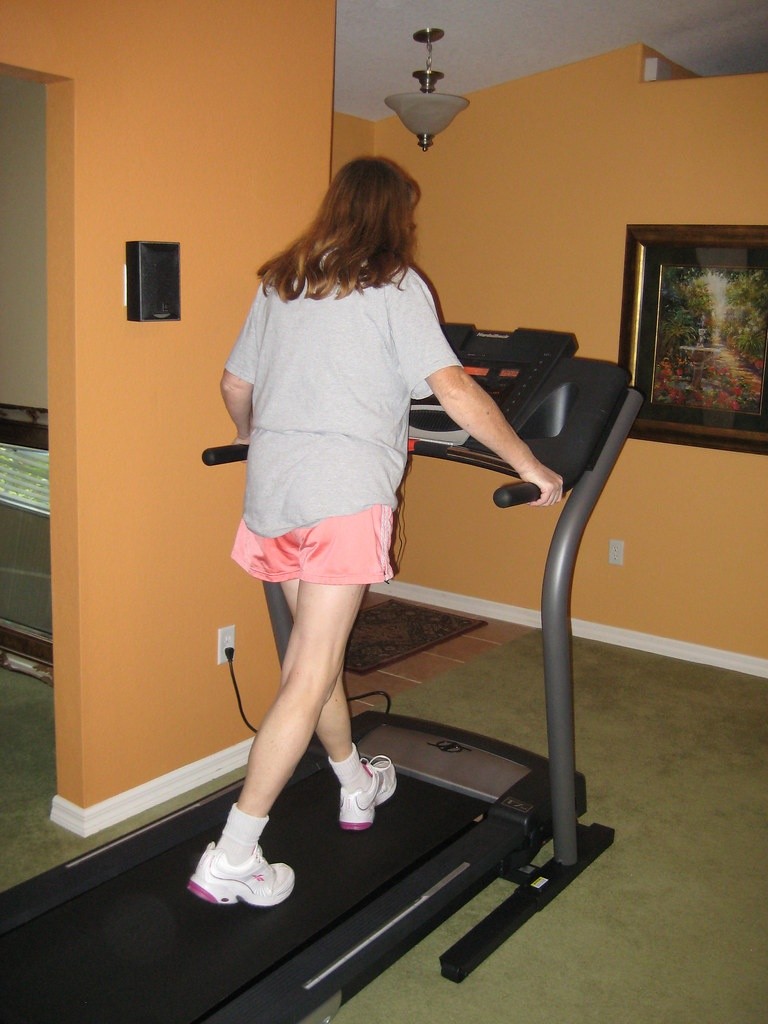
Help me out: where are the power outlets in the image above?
[217,624,235,666]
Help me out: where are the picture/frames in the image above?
[617,224,768,455]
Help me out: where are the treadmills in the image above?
[0,317,646,1024]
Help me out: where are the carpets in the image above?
[343,599,488,677]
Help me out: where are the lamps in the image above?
[383,28,470,153]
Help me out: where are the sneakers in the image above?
[186,841,295,906]
[338,755,397,831]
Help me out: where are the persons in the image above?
[185,157,566,907]
